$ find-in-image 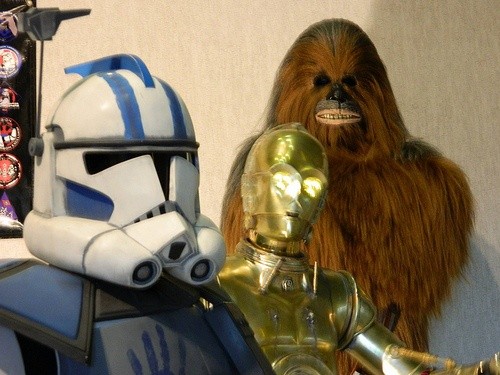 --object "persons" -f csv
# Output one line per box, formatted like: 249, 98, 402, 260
2, 53, 284, 375
199, 123, 498, 374
